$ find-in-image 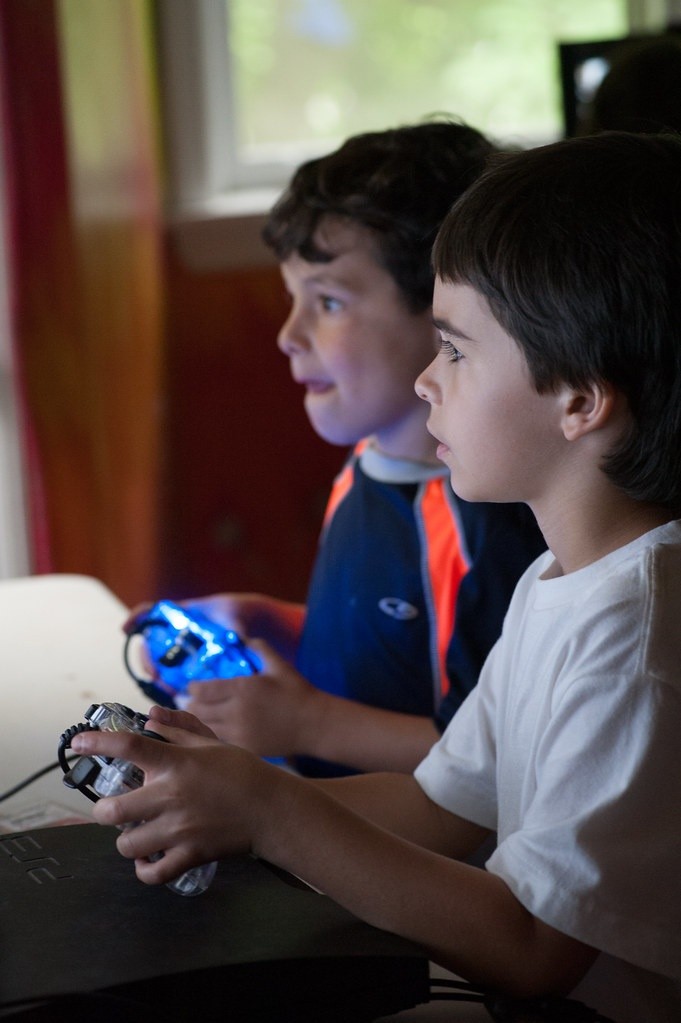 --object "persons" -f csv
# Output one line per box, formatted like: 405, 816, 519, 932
70, 133, 680, 1022
118, 113, 555, 769
